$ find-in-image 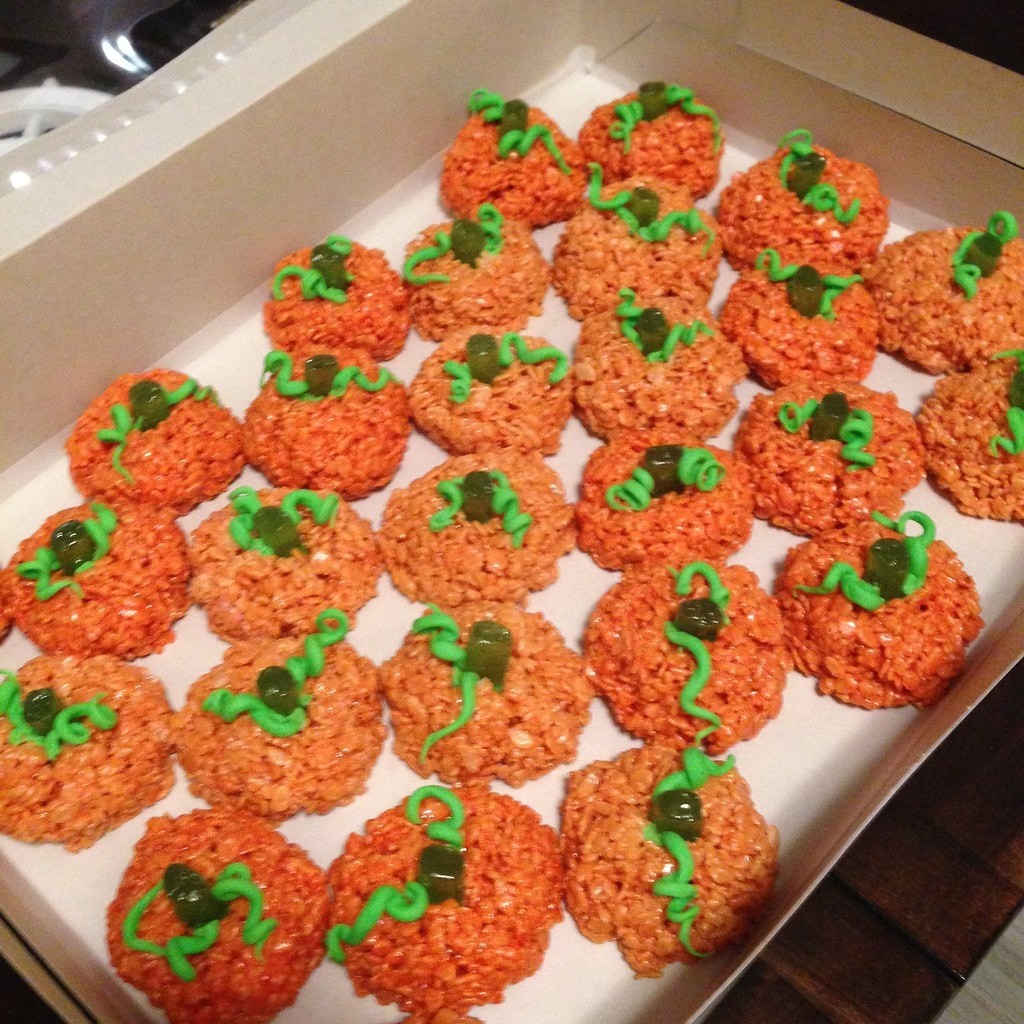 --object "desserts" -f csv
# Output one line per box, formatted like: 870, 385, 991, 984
0, 80, 1024, 1024
